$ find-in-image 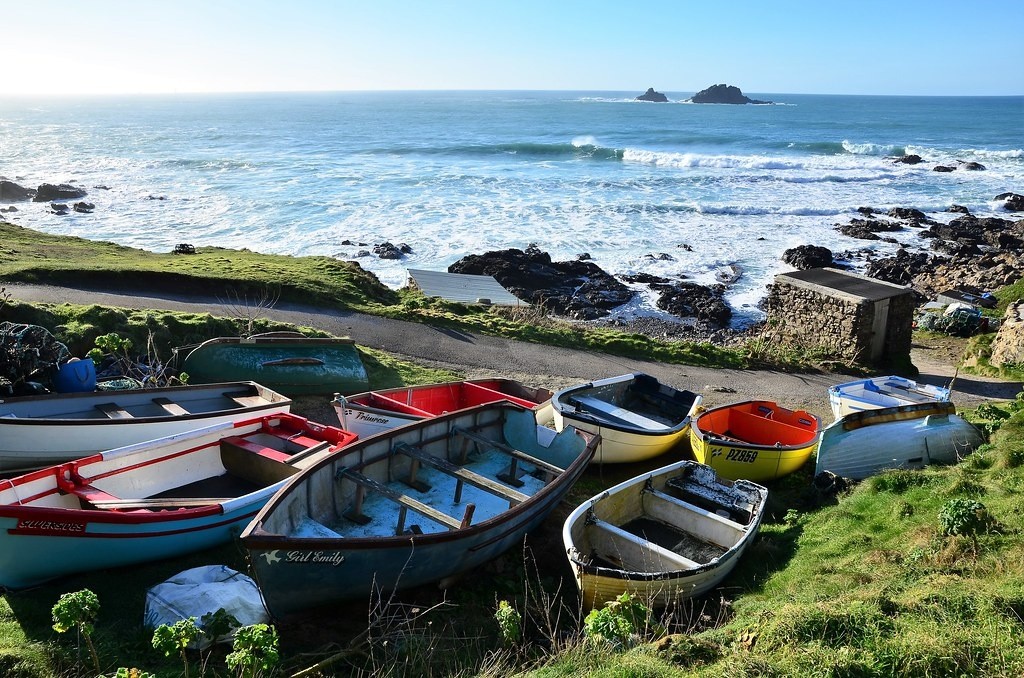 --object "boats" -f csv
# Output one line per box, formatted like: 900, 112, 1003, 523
827, 374, 952, 420
172, 327, 371, 398
331, 377, 556, 442
549, 369, 704, 464
562, 460, 769, 615
239, 398, 603, 631
809, 401, 986, 503
689, 399, 823, 483
0, 379, 293, 463
0, 410, 360, 588
